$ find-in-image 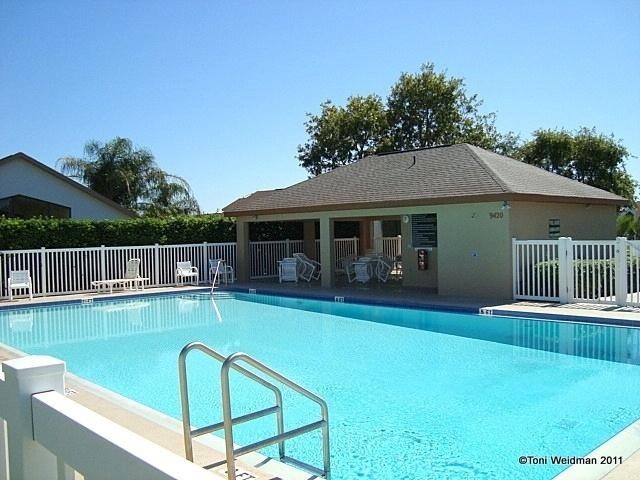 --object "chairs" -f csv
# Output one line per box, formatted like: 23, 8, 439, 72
209, 259, 234, 285
90, 258, 149, 294
7, 270, 33, 301
175, 261, 199, 286
278, 255, 393, 284
